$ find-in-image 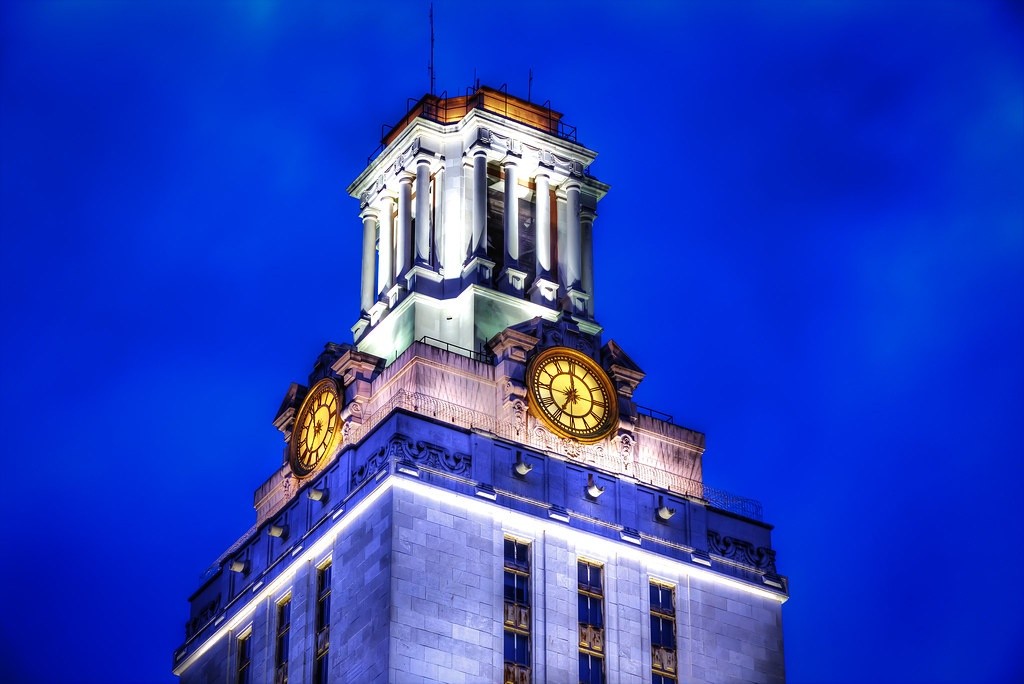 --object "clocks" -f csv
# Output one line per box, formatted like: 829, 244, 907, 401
288, 376, 344, 479
525, 346, 620, 446
